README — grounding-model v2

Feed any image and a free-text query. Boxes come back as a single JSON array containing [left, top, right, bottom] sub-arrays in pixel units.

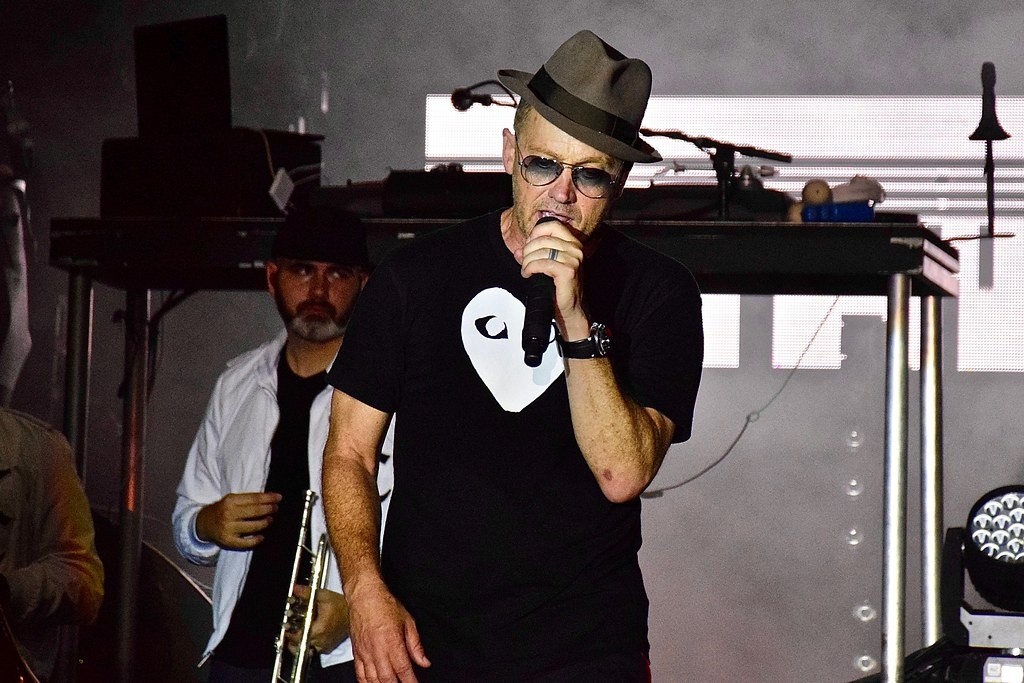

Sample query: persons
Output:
[[323, 29, 704, 683], [174, 204, 397, 683], [0, 411, 110, 683]]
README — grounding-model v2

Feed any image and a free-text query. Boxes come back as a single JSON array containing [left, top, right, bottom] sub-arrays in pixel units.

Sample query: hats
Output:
[[496, 29, 663, 163], [271, 205, 369, 271]]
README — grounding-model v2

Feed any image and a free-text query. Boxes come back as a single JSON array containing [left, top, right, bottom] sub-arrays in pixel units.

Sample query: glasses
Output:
[[514, 134, 628, 199]]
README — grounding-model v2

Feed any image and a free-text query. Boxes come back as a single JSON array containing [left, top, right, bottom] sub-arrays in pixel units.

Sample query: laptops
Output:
[[131, 14, 326, 145]]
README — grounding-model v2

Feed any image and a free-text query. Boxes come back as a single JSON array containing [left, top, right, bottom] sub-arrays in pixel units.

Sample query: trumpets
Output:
[[270, 490, 332, 683]]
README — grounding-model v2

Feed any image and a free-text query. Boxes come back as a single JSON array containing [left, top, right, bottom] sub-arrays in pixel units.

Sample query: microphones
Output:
[[452, 88, 493, 111], [521, 216, 564, 368]]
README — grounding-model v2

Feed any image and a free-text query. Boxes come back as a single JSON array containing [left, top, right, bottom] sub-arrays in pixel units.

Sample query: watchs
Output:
[[553, 322, 614, 360]]
